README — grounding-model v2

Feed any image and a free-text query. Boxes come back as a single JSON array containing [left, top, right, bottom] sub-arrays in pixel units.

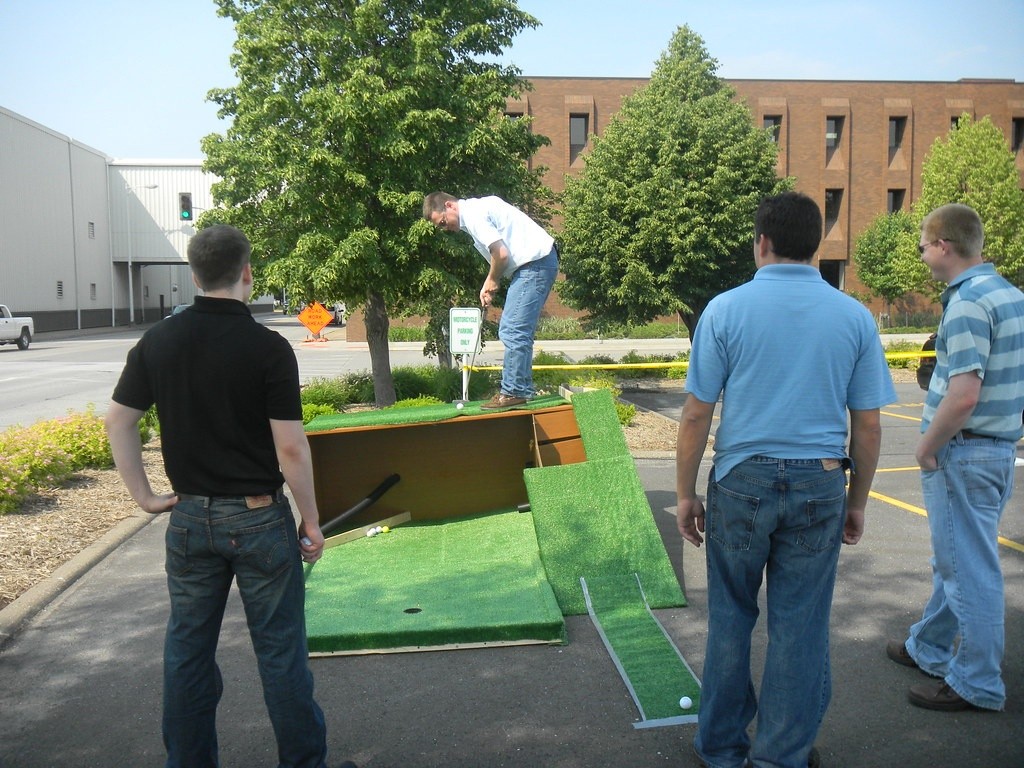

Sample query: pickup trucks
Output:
[[0, 304, 34, 350]]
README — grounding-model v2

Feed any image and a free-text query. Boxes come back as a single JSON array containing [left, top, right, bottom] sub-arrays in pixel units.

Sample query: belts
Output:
[[951, 428, 997, 443], [173, 491, 246, 503]]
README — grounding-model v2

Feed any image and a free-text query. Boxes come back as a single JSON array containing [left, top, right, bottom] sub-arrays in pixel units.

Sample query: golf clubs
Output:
[[450, 301, 489, 407]]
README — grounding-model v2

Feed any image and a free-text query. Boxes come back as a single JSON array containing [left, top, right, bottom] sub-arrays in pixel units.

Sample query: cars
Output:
[[321, 303, 344, 326], [164, 305, 191, 320], [283, 299, 305, 315]]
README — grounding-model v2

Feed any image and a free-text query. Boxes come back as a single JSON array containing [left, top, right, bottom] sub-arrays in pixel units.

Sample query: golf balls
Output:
[[456, 403, 464, 410], [366, 524, 391, 538], [678, 696, 693, 710]]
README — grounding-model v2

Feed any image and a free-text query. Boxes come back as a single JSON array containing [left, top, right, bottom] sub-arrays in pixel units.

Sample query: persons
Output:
[[886, 204, 1024, 712], [423, 191, 559, 410], [676, 192, 896, 768], [105, 224, 357, 768]]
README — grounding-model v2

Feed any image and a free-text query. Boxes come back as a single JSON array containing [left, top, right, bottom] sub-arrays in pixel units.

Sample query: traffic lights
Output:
[[179, 193, 192, 221]]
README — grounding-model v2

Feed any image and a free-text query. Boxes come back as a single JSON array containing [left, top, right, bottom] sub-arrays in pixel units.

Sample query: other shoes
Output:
[[805, 746, 821, 767]]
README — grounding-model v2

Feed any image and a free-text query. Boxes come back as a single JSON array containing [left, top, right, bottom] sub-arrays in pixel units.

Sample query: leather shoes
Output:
[[908, 677, 999, 712], [886, 638, 925, 667]]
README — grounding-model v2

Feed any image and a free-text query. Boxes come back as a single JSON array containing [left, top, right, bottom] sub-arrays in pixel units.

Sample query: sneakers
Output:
[[481, 393, 527, 410]]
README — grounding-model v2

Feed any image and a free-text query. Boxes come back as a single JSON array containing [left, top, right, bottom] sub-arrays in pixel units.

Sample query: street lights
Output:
[[126, 183, 158, 326]]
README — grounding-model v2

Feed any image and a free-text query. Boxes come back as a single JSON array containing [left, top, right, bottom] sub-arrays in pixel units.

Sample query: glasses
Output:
[[918, 238, 959, 253], [434, 209, 446, 229]]
